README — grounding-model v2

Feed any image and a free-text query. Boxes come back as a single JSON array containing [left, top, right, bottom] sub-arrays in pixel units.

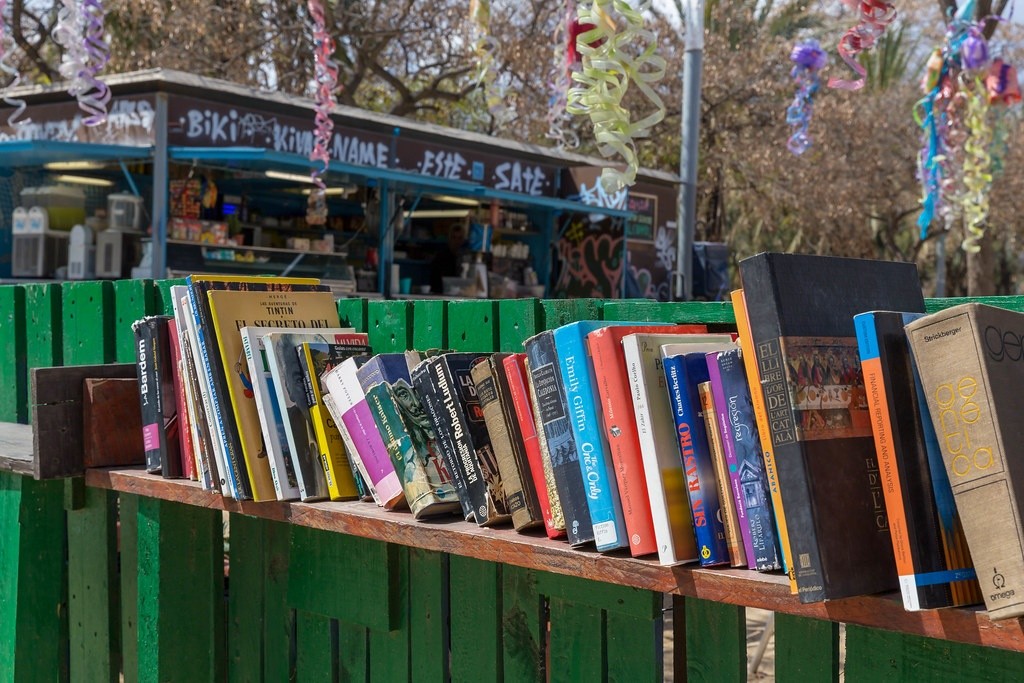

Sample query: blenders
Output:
[[96, 193, 146, 280]]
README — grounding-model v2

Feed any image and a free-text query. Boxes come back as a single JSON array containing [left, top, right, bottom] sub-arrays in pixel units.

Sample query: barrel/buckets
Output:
[[400, 277, 411, 294], [421, 285, 430, 293]]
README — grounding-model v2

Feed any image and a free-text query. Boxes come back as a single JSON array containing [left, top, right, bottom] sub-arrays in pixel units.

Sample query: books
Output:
[[740, 252, 925, 605], [904, 301, 1023, 622], [150, 275, 379, 504], [133, 316, 165, 472], [854, 310, 988, 611], [353, 350, 462, 522], [321, 355, 404, 511], [410, 290, 799, 596]]
